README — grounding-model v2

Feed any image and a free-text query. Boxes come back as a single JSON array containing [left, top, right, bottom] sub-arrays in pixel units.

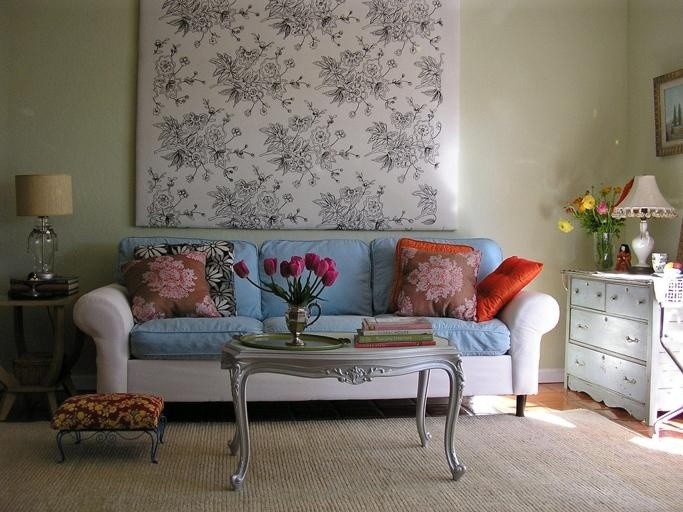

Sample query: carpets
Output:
[[0, 407, 683, 512]]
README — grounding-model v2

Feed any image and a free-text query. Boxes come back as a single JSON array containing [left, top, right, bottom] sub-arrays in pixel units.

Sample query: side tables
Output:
[[0, 291, 85, 420]]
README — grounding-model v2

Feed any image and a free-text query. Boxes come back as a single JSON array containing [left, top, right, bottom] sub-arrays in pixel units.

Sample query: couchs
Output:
[[73, 236, 561, 417]]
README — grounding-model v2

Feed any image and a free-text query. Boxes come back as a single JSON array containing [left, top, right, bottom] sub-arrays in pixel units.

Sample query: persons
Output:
[[616, 244, 632, 272]]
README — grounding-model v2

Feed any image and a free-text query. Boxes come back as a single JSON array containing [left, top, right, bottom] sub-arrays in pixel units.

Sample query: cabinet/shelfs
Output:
[[561, 267, 682, 427]]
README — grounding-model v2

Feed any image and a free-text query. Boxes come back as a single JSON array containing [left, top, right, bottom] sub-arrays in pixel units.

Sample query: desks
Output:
[[221, 332, 467, 491]]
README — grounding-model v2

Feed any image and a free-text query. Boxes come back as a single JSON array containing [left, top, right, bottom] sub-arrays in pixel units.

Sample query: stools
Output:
[[51, 392, 167, 464]]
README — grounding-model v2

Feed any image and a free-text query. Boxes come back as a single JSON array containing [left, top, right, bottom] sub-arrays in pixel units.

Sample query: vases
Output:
[[284, 302, 321, 346], [592, 233, 617, 272]]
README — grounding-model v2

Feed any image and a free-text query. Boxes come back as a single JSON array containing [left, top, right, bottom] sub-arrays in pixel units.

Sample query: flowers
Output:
[[557, 183, 626, 239], [233, 253, 339, 306]]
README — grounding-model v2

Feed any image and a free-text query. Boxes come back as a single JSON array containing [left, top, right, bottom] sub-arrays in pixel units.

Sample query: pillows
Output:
[[133, 240, 238, 317], [116, 250, 223, 324], [385, 238, 544, 323]]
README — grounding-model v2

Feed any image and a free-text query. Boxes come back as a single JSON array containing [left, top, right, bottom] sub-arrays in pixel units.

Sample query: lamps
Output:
[[8, 173, 80, 280], [611, 175, 679, 268]]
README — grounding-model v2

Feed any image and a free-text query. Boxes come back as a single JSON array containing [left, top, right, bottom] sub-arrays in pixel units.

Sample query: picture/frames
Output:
[[653, 69, 683, 157]]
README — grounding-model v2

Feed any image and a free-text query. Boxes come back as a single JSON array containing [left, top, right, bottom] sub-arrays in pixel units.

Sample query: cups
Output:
[[652, 253, 668, 273]]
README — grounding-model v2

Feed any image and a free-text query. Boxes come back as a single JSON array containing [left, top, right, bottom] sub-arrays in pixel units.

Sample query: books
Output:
[[354, 317, 436, 348]]
